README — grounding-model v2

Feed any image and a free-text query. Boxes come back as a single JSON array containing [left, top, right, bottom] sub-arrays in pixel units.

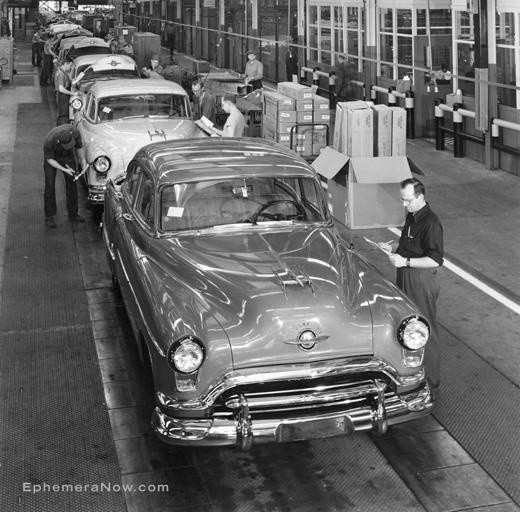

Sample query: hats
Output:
[[58, 130, 76, 150]]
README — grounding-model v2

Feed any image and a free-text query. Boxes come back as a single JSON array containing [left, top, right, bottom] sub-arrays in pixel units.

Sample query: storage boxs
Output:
[[260, 81, 427, 231]]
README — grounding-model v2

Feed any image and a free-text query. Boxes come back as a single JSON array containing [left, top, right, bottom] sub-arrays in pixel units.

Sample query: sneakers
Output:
[[45, 217, 55, 227], [68, 214, 85, 222]]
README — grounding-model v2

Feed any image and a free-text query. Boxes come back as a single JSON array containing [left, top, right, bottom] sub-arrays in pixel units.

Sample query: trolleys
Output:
[[289, 124, 329, 165], [216, 83, 254, 126]]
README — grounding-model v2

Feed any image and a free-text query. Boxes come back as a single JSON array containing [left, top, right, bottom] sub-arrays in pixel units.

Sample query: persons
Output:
[[241, 50, 263, 93], [333, 52, 358, 123], [44, 124, 87, 228], [32, 27, 219, 129], [210, 93, 246, 137], [378, 178, 444, 338]]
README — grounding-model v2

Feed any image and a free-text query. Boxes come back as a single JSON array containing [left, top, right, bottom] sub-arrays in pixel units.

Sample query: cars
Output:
[[232, 6, 294, 61], [67, 53, 143, 121], [72, 78, 211, 224], [102, 137, 435, 453], [37, 10, 108, 89]]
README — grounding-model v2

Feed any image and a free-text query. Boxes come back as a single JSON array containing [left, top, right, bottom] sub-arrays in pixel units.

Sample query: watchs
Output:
[[405, 257, 410, 267]]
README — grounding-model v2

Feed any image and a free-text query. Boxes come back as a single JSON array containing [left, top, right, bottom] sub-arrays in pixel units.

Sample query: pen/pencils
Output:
[[385, 240, 394, 244]]
